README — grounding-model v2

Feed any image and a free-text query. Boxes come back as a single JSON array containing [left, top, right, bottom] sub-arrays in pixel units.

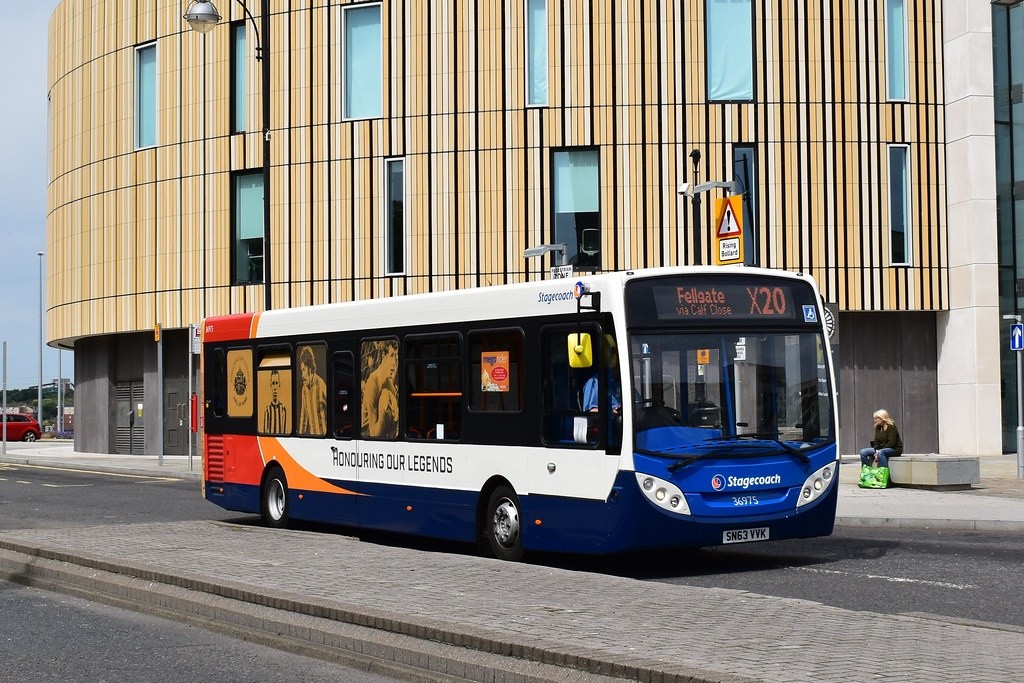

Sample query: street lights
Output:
[[183, 0, 273, 312], [38, 252, 45, 435]]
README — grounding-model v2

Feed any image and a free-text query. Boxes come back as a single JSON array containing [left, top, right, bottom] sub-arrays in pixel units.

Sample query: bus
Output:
[[198, 267, 845, 569]]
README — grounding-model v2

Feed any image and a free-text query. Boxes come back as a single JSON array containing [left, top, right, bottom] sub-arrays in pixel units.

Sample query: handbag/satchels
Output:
[[858, 464, 891, 489]]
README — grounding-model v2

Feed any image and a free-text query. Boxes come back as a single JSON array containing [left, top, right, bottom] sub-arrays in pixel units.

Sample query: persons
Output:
[[361, 341, 399, 438], [361, 349, 379, 391], [264, 370, 287, 434], [583, 346, 643, 439], [299, 346, 327, 435], [860, 408, 904, 489]]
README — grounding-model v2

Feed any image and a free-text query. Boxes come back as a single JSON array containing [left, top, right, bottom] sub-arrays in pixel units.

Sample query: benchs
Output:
[[873, 453, 980, 491]]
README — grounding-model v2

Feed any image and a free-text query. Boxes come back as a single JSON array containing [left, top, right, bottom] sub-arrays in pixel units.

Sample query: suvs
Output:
[[0, 412, 42, 443]]
